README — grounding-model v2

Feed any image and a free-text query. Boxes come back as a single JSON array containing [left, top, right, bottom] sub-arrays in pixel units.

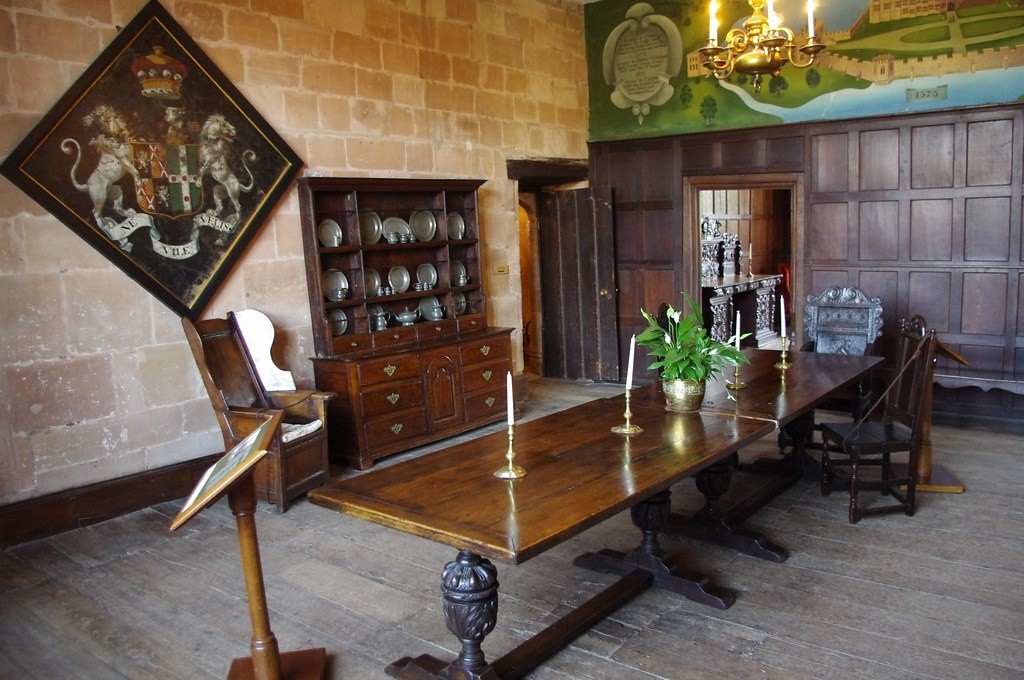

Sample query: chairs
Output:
[[180, 309, 338, 513], [777, 285, 884, 453], [820, 317, 937, 524]]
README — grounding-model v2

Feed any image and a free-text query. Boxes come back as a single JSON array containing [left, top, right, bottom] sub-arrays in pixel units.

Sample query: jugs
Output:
[[458, 275, 469, 286], [431, 304, 443, 320]]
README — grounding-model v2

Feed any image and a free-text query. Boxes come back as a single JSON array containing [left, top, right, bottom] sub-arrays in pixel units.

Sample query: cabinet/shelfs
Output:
[[295, 175, 518, 469], [699, 217, 785, 352]]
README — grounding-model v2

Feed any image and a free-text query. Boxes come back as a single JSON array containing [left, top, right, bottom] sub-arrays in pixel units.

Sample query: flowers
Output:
[[633, 290, 753, 390]]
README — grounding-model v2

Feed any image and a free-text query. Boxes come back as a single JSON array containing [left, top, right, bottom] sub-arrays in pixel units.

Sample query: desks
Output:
[[306, 352, 883, 678]]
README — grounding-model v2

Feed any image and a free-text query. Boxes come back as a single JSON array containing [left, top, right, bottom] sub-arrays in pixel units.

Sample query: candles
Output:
[[699, 0, 827, 93], [505, 369, 516, 429], [624, 334, 637, 391]]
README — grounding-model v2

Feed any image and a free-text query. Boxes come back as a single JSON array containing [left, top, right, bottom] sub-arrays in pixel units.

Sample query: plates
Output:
[[416, 263, 437, 286], [322, 272, 349, 301], [409, 205, 436, 241], [364, 267, 381, 295], [450, 260, 466, 284], [318, 218, 343, 247], [447, 211, 465, 240], [326, 308, 348, 336], [452, 292, 466, 316], [358, 208, 382, 244], [419, 296, 439, 320], [388, 266, 410, 293], [382, 217, 412, 241], [366, 304, 383, 330]]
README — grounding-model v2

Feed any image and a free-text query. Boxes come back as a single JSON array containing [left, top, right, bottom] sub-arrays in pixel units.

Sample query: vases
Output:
[[660, 371, 707, 414]]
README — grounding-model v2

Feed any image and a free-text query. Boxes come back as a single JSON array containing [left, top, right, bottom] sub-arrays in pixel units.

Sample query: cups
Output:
[[384, 286, 391, 296], [391, 287, 396, 294], [409, 233, 416, 243], [376, 287, 384, 296], [387, 232, 398, 244], [331, 236, 342, 248], [400, 234, 407, 243], [422, 282, 428, 290], [429, 283, 433, 290]]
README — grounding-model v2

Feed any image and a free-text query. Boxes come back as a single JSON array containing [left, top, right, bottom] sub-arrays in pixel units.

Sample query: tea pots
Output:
[[413, 282, 422, 291], [374, 311, 391, 331], [392, 306, 422, 327], [335, 287, 348, 301]]
